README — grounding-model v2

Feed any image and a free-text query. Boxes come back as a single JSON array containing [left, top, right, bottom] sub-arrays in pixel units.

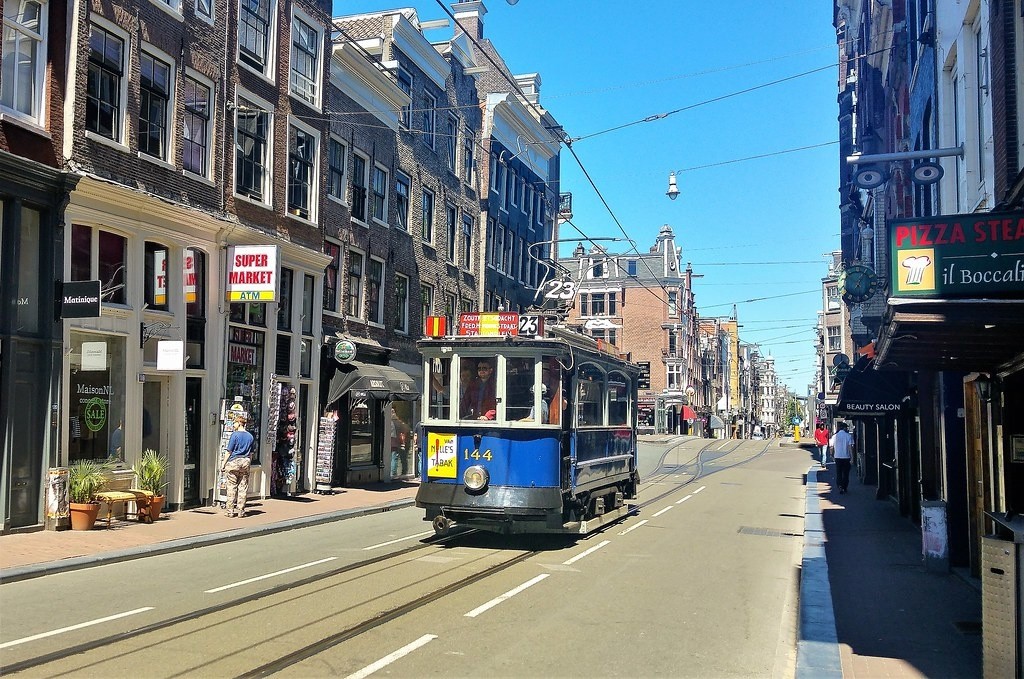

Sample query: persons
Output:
[[519, 383, 550, 424], [389, 409, 424, 479], [430, 363, 476, 411], [111, 419, 122, 459], [457, 359, 498, 422], [828, 423, 855, 494], [814, 424, 829, 468], [220, 414, 254, 517]]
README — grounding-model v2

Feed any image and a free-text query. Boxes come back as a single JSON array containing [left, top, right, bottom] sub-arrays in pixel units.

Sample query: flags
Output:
[[324, 355, 420, 410]]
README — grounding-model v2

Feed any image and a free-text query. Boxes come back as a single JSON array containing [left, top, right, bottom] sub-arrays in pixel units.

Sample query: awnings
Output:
[[682, 406, 698, 420], [708, 415, 725, 429], [836, 353, 920, 419]]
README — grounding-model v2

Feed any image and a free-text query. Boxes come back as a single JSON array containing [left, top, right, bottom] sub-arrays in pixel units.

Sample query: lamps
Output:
[[666, 170, 681, 201], [974, 373, 992, 402]]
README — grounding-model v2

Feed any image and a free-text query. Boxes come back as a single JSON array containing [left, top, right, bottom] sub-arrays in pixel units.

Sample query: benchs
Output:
[[94, 488, 155, 531]]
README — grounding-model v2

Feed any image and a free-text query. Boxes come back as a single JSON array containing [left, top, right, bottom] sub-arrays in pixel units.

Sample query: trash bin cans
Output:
[[978, 510, 1024, 679]]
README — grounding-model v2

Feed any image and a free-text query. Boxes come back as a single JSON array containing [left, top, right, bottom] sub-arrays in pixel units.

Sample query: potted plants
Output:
[[65, 457, 115, 530], [120, 448, 174, 520]]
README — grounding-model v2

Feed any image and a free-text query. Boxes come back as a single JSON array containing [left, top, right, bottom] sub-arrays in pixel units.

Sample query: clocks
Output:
[[837, 265, 878, 302]]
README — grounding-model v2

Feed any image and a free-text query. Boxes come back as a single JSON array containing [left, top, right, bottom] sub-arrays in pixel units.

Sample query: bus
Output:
[[414, 237, 646, 539]]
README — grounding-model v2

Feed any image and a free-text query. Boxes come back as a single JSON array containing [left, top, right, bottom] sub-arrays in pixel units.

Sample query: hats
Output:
[[841, 423, 849, 429], [529, 383, 547, 394]]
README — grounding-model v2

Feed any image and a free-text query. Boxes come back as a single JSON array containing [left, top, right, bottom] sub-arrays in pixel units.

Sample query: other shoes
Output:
[[224, 512, 234, 517], [238, 513, 247, 518], [839, 487, 845, 494], [821, 464, 825, 468]]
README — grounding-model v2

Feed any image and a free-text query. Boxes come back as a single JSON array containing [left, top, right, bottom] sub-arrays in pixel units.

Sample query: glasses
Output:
[[477, 366, 492, 372]]
